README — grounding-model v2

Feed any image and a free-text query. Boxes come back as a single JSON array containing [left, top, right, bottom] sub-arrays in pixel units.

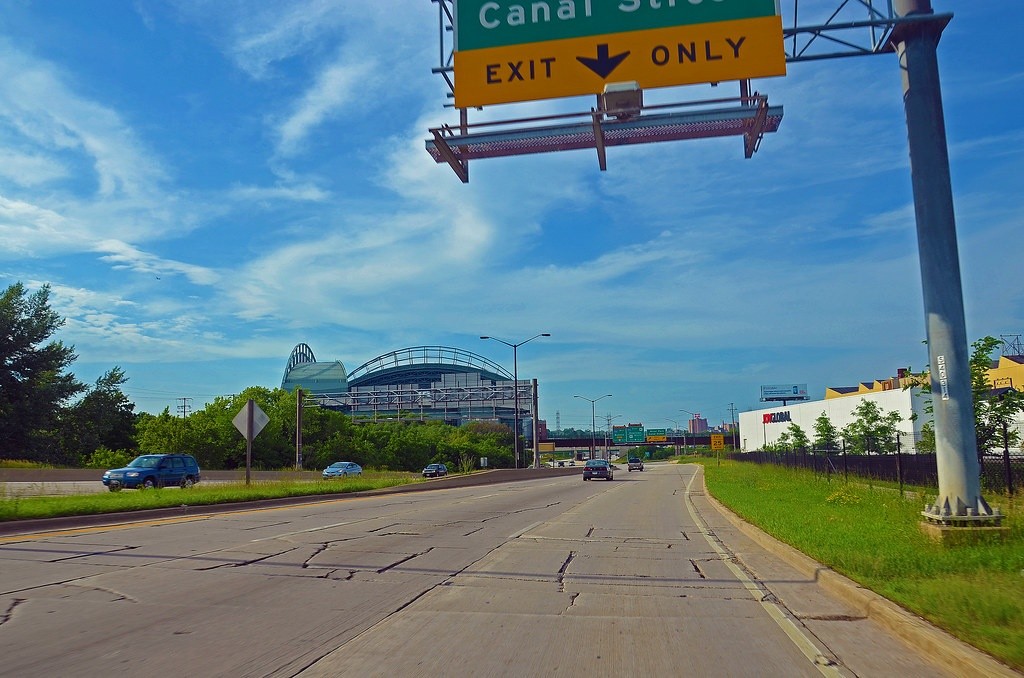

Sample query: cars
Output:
[[558, 462, 565, 467], [628, 457, 644, 472], [322, 462, 363, 480], [582, 459, 613, 481], [569, 460, 576, 466], [422, 464, 448, 478]]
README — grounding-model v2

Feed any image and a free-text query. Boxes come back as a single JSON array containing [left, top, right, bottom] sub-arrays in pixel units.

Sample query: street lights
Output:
[[596, 414, 623, 468], [574, 394, 612, 459], [679, 409, 696, 447], [665, 417, 678, 457], [480, 333, 551, 467]]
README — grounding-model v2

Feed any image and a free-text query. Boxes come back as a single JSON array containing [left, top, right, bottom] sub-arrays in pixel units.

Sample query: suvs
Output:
[[101, 452, 201, 492]]
